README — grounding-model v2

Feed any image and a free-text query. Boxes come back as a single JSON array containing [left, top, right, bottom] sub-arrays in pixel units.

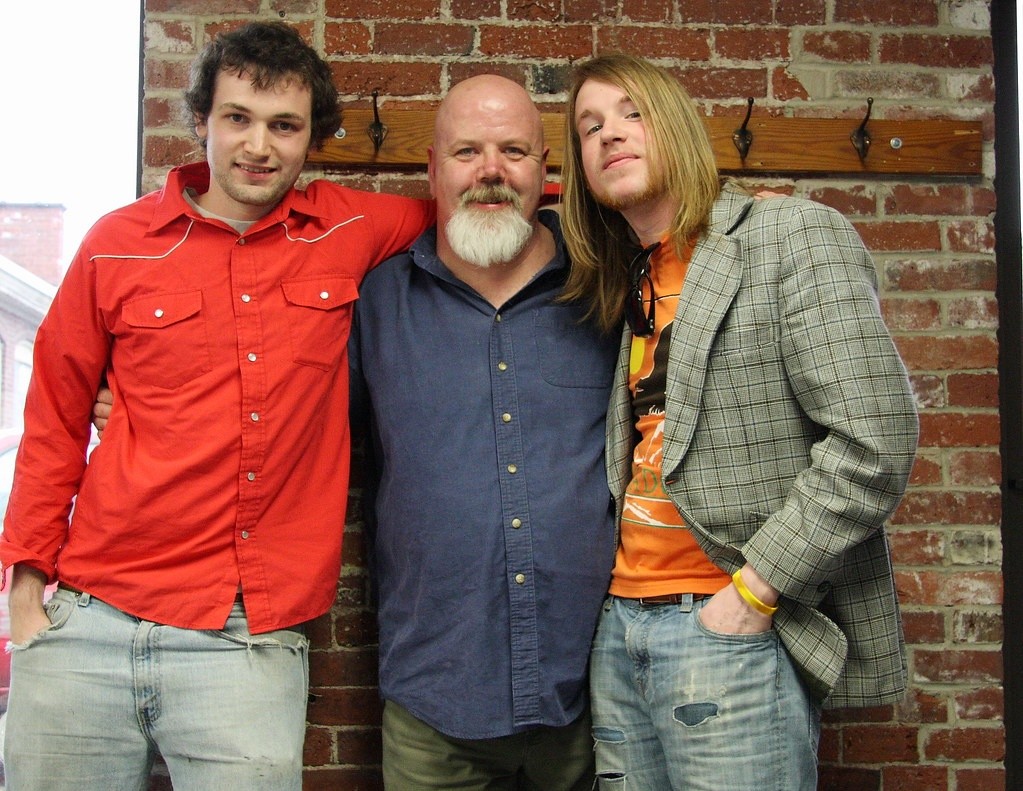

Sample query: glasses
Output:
[[622, 242, 662, 337]]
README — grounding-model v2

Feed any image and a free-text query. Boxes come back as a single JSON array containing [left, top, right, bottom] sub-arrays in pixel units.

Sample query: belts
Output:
[[615, 592, 715, 606]]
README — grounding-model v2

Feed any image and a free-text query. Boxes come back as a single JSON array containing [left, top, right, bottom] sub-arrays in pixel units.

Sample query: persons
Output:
[[558, 54, 919, 791], [92, 73, 779, 791], [0, 20, 567, 791]]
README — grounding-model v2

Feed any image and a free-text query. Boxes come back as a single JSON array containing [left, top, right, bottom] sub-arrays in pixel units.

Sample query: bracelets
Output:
[[731, 569, 779, 616]]
